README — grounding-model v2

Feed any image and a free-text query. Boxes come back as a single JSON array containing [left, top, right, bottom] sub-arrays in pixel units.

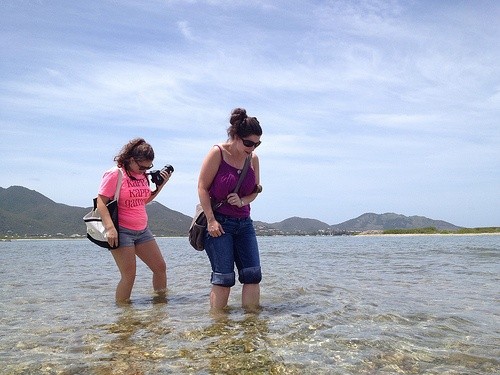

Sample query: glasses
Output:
[[135, 161, 153, 170], [240, 137, 261, 148]]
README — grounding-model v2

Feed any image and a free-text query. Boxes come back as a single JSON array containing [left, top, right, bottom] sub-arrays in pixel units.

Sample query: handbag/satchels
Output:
[[82, 169, 122, 249], [189, 200, 224, 251]]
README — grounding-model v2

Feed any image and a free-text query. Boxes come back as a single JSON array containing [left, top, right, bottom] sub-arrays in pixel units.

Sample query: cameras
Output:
[[150, 165, 174, 186]]
